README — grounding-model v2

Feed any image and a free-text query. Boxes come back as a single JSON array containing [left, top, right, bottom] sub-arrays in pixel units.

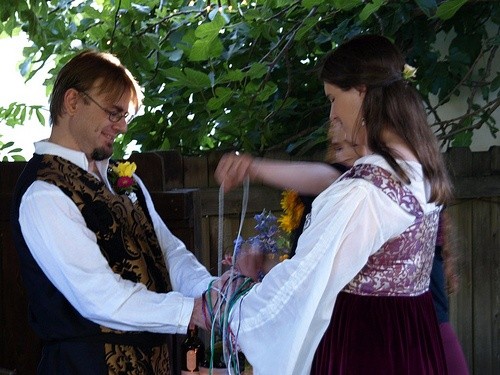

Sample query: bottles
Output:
[[185, 323, 201, 373]]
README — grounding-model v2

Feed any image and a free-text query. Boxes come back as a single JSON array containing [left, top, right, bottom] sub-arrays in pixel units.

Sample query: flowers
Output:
[[243, 188, 303, 262], [111, 161, 137, 194]]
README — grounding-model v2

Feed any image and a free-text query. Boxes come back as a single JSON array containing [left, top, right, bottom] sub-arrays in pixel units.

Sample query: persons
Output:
[[10, 34, 467, 375]]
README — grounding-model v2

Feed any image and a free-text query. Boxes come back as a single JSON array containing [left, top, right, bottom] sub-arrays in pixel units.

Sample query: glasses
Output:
[[80, 90, 134, 125]]
